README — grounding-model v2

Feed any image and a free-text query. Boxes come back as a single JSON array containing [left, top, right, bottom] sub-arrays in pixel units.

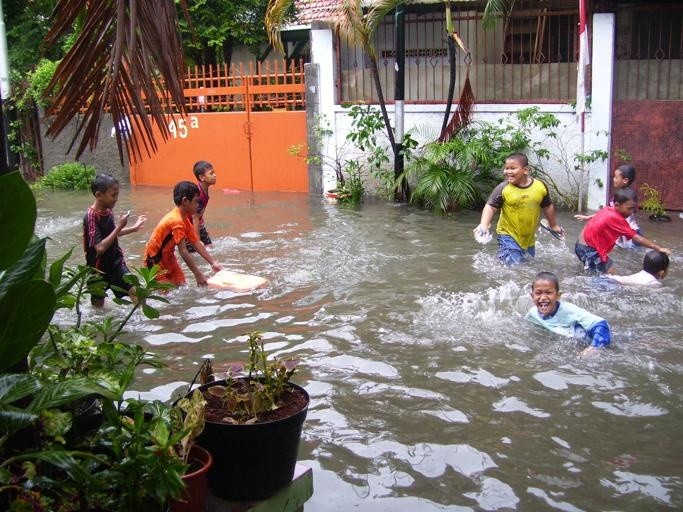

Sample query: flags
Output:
[[574, 1, 591, 123]]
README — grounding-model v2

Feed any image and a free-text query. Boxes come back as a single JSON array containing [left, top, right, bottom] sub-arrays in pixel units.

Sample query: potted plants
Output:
[[178, 330, 311, 500], [137, 389, 214, 510], [289, 104, 385, 206], [0, 170, 172, 509]]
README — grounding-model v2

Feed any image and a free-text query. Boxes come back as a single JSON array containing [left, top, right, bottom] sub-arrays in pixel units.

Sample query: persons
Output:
[[82, 174, 147, 310], [592, 250, 670, 295], [575, 188, 673, 275], [525, 272, 612, 360], [472, 153, 566, 266], [142, 180, 225, 298], [184, 160, 216, 253], [573, 164, 644, 248]]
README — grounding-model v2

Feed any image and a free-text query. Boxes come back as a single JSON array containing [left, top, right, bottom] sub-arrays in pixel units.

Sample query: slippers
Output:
[[472, 223, 490, 243], [540, 218, 566, 241]]
